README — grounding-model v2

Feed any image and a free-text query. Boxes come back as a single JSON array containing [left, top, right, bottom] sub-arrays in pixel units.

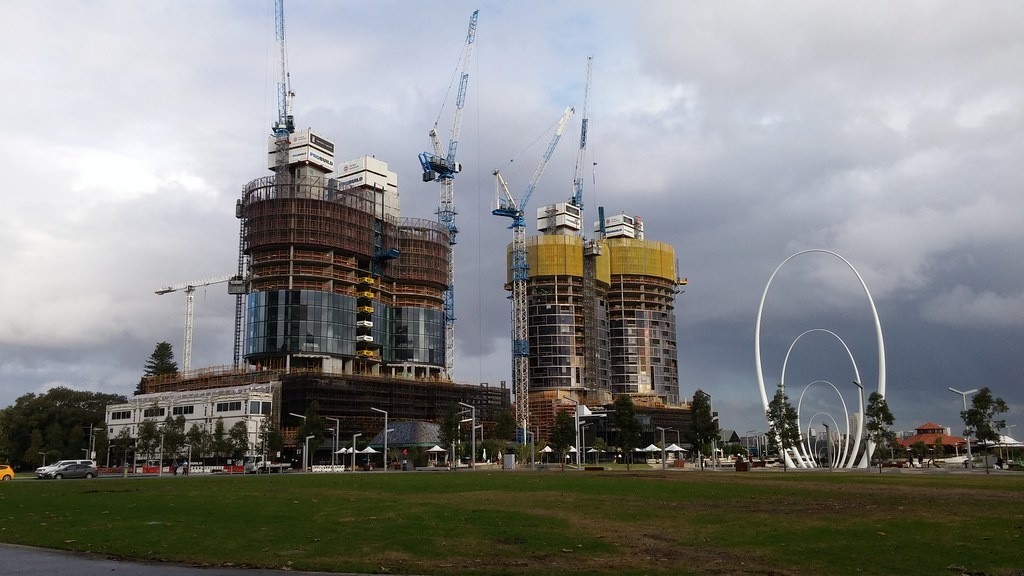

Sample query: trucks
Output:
[[245, 454, 292, 474]]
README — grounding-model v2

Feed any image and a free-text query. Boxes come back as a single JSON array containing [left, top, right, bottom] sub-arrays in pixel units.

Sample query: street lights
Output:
[[305, 435, 315, 472], [81, 448, 88, 460], [656, 426, 666, 473], [458, 401, 476, 472], [328, 428, 335, 471], [184, 443, 192, 476], [289, 412, 307, 427], [326, 417, 339, 465], [352, 433, 363, 471], [852, 379, 871, 469], [948, 387, 978, 471], [370, 407, 387, 470], [38, 452, 45, 478], [107, 444, 117, 468], [250, 419, 258, 454], [821, 421, 833, 472]]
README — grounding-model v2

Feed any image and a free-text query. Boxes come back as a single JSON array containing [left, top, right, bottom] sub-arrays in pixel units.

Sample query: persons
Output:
[[816, 458, 821, 468], [172, 458, 178, 476], [182, 460, 188, 475], [918, 453, 923, 464], [996, 457, 1003, 467], [737, 453, 743, 462], [716, 456, 721, 468], [909, 454, 915, 468], [821, 457, 824, 465], [749, 453, 753, 468]]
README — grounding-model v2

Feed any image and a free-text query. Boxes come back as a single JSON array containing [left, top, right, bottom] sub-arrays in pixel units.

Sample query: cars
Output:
[[0, 465, 15, 481], [45, 464, 98, 480]]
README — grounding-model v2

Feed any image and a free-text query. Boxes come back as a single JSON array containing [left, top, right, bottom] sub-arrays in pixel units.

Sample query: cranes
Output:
[[274, 0, 296, 198], [155, 275, 233, 392], [418, 9, 478, 383], [490, 107, 575, 445], [567, 56, 593, 208]]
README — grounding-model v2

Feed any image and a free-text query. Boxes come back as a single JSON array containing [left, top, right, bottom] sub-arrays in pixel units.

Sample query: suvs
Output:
[[35, 459, 97, 479]]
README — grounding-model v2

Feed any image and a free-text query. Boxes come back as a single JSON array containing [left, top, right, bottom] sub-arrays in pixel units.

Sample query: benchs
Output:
[[705, 460, 736, 468]]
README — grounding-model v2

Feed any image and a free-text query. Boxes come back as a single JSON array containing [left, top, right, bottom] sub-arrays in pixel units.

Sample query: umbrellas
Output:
[[977, 435, 1024, 463], [630, 443, 688, 459], [565, 446, 582, 463], [333, 446, 382, 465], [586, 448, 606, 460], [425, 445, 448, 451], [539, 446, 555, 463]]
